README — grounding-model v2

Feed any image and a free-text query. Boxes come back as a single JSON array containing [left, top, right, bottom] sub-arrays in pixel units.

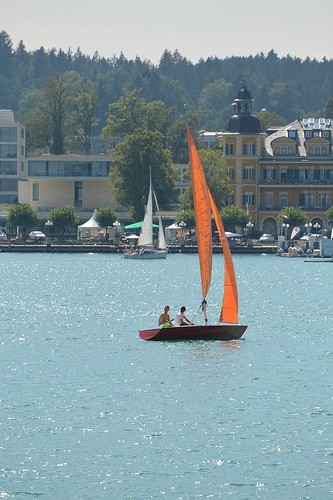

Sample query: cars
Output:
[[28, 231, 46, 241], [256, 234, 275, 245]]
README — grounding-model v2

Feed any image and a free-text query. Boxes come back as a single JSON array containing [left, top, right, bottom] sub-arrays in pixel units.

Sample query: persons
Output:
[[275, 247, 284, 256], [176, 306, 194, 326], [288, 246, 304, 256], [158, 306, 174, 327], [116, 240, 121, 246], [123, 247, 145, 256], [306, 248, 313, 255]]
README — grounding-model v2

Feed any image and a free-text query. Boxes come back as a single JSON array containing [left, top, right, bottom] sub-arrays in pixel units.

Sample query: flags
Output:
[[291, 227, 300, 240]]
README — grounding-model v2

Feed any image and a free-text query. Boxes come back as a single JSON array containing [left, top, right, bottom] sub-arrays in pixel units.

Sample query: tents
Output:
[[164, 222, 185, 242], [77, 208, 114, 243], [123, 221, 159, 244]]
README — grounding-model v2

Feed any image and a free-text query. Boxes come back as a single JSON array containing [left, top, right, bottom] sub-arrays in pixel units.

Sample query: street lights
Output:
[[113, 220, 121, 246], [304, 222, 313, 236], [313, 222, 321, 237], [281, 222, 290, 240], [178, 221, 187, 247], [45, 220, 53, 247], [246, 221, 254, 248]]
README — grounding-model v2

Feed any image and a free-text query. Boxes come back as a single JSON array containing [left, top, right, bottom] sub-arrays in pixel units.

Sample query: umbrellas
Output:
[[125, 234, 140, 239], [217, 231, 243, 239]]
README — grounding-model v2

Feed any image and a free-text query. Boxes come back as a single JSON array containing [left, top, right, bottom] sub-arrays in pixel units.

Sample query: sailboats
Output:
[[137, 123, 248, 342], [122, 165, 168, 260]]
